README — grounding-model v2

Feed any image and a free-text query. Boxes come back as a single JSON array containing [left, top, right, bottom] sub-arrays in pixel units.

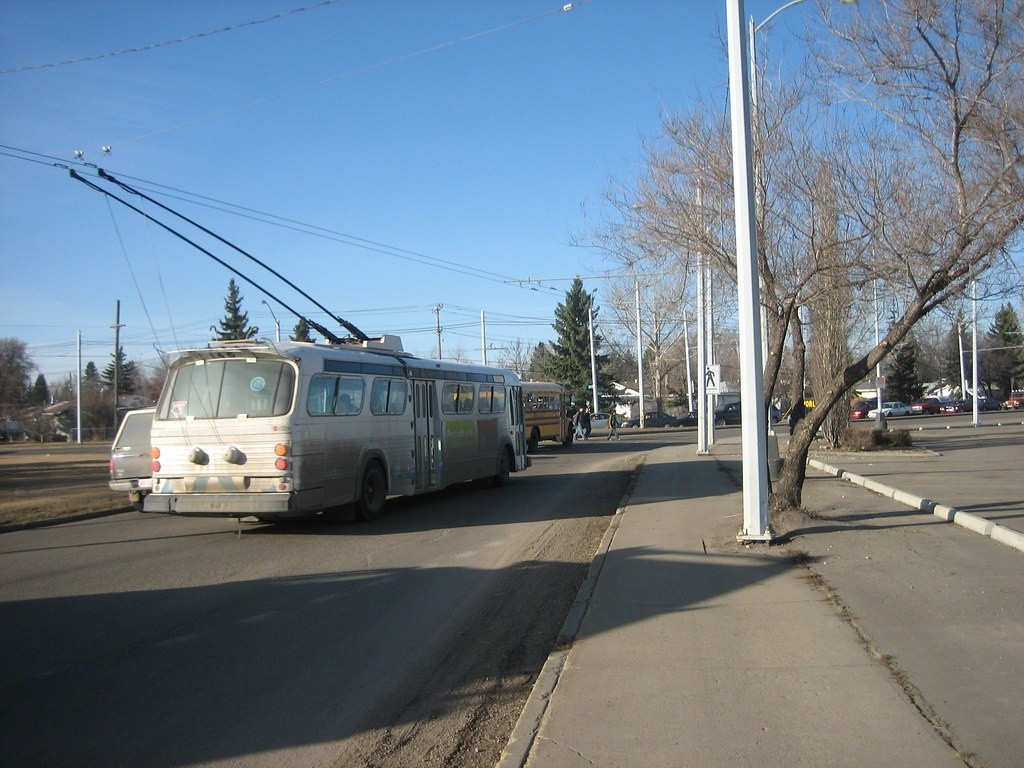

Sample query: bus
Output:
[[453, 380, 575, 455], [68, 163, 534, 524]]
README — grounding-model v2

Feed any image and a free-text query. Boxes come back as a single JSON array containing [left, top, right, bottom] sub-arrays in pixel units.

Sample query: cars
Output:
[[868, 401, 914, 419], [938, 389, 1024, 416], [621, 410, 678, 430], [850, 400, 875, 420], [671, 411, 698, 428], [712, 399, 781, 427], [578, 413, 623, 438], [909, 396, 951, 415]]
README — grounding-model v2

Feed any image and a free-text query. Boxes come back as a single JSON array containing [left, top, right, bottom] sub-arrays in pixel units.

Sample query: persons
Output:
[[787, 398, 806, 446], [606, 410, 621, 441], [572, 400, 596, 441], [337, 394, 358, 413], [442, 396, 501, 412]]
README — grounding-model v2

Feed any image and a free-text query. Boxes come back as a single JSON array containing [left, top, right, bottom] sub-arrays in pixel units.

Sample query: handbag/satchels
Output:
[[607, 421, 610, 429]]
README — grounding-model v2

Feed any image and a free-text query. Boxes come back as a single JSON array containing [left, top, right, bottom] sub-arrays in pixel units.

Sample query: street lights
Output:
[[588, 287, 598, 418], [261, 299, 281, 341], [634, 179, 710, 456]]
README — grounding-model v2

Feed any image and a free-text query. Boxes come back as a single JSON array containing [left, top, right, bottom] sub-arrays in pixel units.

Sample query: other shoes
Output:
[[617, 438, 621, 441], [607, 438, 611, 441]]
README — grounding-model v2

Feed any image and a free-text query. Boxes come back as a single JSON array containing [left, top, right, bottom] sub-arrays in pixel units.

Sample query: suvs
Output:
[[108, 409, 158, 513]]
